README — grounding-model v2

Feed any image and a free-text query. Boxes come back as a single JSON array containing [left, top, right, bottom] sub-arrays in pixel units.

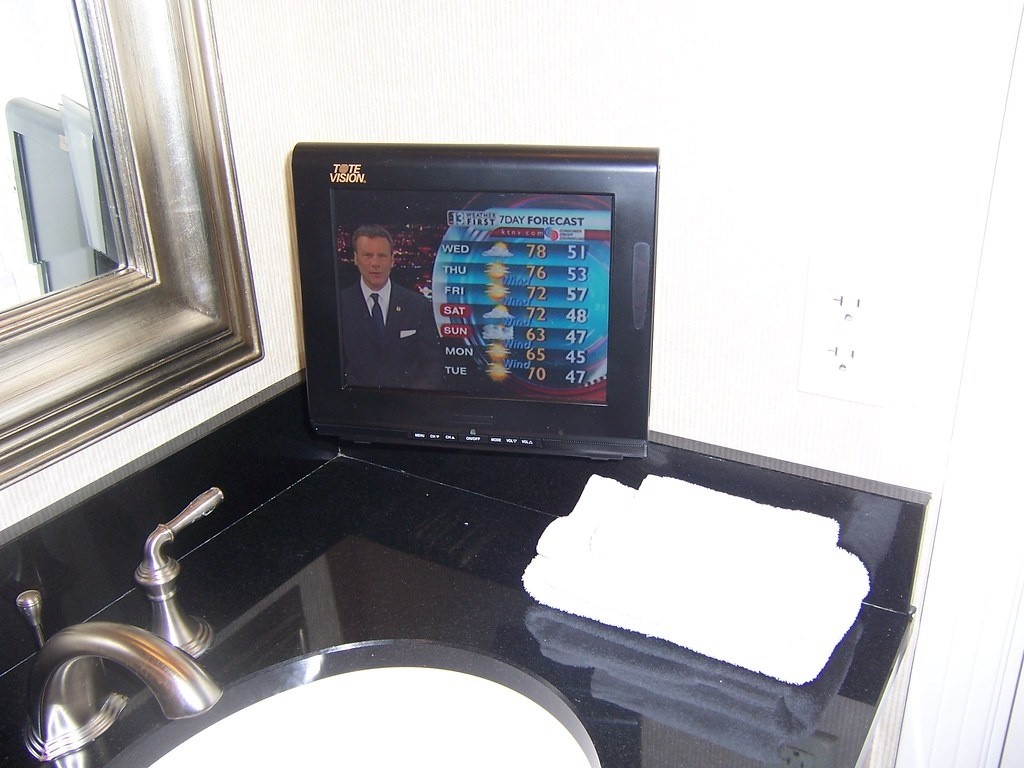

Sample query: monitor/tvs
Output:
[[289, 142, 658, 458]]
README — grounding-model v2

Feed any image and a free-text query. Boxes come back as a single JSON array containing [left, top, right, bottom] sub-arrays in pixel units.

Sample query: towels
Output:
[[520, 472, 870, 685]]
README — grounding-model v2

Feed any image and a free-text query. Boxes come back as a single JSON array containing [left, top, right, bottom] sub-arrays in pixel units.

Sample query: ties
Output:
[[370, 293, 384, 341]]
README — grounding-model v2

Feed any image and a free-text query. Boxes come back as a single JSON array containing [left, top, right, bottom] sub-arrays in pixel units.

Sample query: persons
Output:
[[338, 226, 443, 390]]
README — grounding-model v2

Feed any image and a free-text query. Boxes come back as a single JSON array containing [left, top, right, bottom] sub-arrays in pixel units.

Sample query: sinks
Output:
[[104, 639, 603, 768]]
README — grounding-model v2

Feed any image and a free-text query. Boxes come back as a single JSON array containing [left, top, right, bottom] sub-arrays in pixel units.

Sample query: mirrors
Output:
[[0, 0, 265, 486]]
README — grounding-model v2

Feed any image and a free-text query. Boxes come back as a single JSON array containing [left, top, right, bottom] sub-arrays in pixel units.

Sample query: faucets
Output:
[[23, 622, 225, 761]]
